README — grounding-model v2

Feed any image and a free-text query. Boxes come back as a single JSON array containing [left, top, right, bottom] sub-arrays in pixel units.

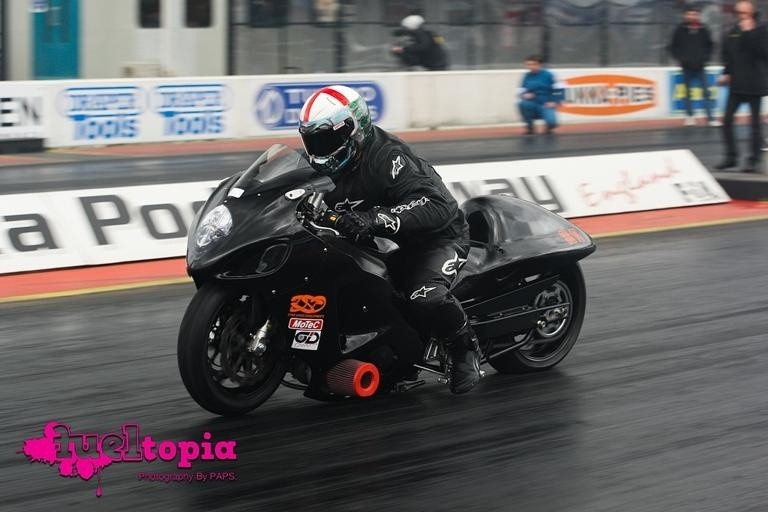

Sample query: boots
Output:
[[444, 316, 482, 393]]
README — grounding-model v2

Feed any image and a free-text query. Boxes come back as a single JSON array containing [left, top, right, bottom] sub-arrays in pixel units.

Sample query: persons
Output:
[[666, 8, 722, 127], [385, 13, 449, 72], [514, 53, 559, 135], [297, 81, 487, 395], [712, 2, 767, 175]]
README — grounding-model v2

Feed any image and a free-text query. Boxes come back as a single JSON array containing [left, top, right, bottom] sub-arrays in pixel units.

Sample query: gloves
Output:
[[337, 210, 375, 240]]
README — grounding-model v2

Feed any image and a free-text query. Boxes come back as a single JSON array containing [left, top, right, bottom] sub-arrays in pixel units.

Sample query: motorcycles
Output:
[[174, 140, 600, 418]]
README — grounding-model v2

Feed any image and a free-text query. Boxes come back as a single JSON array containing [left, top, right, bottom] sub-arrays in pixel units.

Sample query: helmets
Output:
[[299, 84, 374, 179], [401, 13, 424, 29]]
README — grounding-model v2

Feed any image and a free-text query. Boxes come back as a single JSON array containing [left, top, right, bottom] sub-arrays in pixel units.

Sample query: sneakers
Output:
[[685, 116, 696, 126], [714, 160, 737, 170], [743, 157, 759, 170], [708, 118, 724, 127]]
